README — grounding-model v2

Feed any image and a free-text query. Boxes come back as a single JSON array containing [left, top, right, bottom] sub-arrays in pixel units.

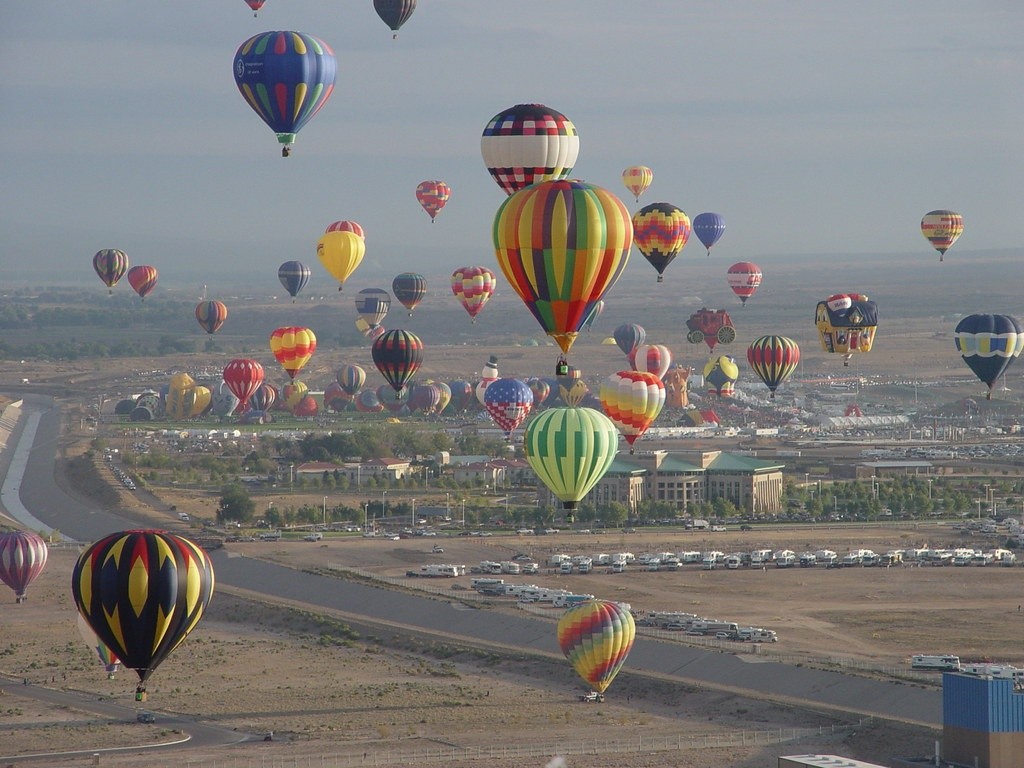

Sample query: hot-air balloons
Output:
[[88, 306, 801, 421], [91, 249, 130, 295], [622, 166, 653, 203], [415, 178, 451, 223], [276, 260, 311, 303], [245, 0, 268, 18], [316, 231, 367, 292], [481, 377, 533, 442], [481, 102, 581, 202], [693, 212, 726, 256], [492, 178, 639, 377], [231, 31, 338, 159], [595, 369, 668, 453], [727, 262, 762, 308], [325, 220, 365, 248], [73, 529, 215, 703], [193, 300, 227, 332], [127, 266, 159, 304], [75, 611, 120, 680], [523, 404, 620, 523], [390, 273, 428, 317], [955, 314, 1024, 401], [814, 293, 879, 366], [355, 288, 391, 338], [0, 530, 48, 605], [374, 0, 418, 40], [449, 266, 497, 323], [557, 598, 637, 703], [631, 202, 691, 283], [920, 210, 964, 262]]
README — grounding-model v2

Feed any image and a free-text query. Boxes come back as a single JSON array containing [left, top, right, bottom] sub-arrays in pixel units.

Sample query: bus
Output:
[[416, 515, 1023, 683]]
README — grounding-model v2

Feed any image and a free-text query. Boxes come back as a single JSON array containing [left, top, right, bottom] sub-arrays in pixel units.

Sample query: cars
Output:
[[162, 504, 413, 548], [99, 445, 136, 492]]
